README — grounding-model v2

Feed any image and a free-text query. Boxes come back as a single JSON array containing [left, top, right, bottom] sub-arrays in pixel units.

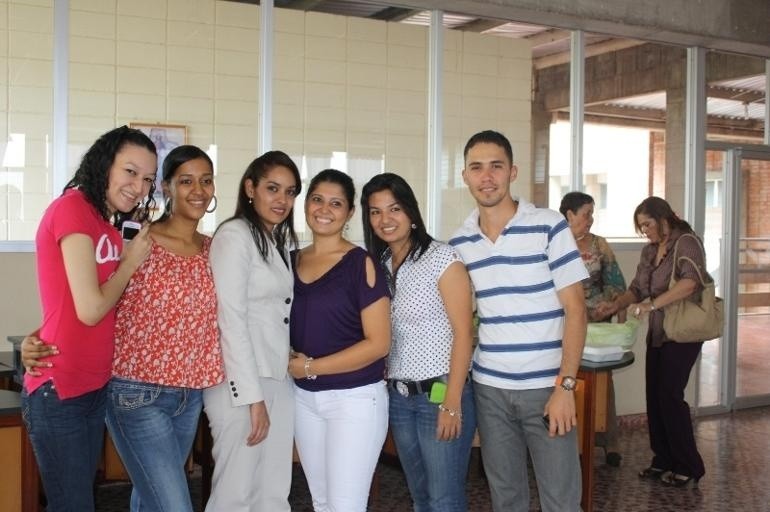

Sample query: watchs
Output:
[[554, 374, 578, 392]]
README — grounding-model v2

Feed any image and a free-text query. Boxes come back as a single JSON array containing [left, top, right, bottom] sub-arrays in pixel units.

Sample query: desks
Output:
[[0, 351, 16, 389], [0, 389, 38, 511], [7, 335, 25, 378], [573, 352, 635, 511]]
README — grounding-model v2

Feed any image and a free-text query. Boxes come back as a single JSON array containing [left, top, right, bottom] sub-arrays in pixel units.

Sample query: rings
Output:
[[634, 306, 642, 317]]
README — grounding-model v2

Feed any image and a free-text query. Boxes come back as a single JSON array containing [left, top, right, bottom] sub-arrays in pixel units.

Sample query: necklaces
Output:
[[575, 234, 587, 241]]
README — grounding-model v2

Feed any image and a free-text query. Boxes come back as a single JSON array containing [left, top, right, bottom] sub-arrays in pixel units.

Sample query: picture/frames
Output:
[[128, 121, 189, 211]]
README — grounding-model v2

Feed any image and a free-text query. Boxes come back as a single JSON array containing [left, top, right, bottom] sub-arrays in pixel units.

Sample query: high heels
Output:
[[639, 467, 666, 480], [666, 464, 705, 490]]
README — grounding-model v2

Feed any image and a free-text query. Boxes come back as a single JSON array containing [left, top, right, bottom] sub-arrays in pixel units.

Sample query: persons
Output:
[[287, 168, 393, 510], [20, 124, 159, 512], [446, 129, 591, 512], [198, 149, 303, 510], [19, 145, 219, 512], [359, 172, 478, 511], [589, 195, 707, 489], [556, 190, 628, 468]]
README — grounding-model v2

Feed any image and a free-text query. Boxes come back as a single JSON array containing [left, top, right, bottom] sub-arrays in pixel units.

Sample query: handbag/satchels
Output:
[[662, 273, 724, 343]]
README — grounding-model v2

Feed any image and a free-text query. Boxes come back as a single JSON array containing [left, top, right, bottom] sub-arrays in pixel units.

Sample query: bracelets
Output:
[[303, 356, 318, 381], [437, 404, 464, 419], [650, 298, 657, 312]]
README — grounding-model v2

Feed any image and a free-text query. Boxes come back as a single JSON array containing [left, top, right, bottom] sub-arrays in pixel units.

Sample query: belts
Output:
[[388, 374, 448, 398]]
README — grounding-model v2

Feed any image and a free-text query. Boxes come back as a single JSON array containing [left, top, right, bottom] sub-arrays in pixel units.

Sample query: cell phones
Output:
[[122, 221, 142, 241], [430, 381, 446, 405]]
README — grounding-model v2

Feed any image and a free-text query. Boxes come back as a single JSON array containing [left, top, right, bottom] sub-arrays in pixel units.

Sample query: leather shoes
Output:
[[606, 452, 621, 469]]
